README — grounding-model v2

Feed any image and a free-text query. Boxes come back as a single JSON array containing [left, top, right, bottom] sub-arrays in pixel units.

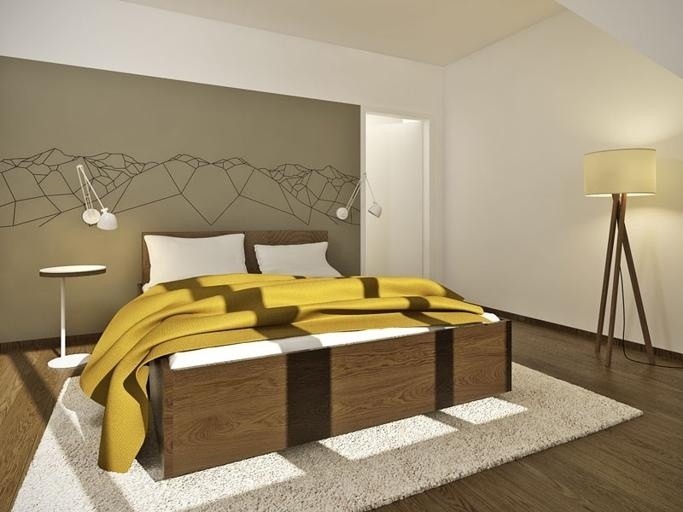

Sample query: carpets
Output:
[[10, 360, 644, 512]]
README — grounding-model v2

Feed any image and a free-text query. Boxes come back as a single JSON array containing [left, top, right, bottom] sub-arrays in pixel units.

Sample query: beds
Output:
[[137, 232, 512, 480]]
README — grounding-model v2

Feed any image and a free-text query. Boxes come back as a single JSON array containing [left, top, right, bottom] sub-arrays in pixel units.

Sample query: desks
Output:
[[39, 265, 106, 369]]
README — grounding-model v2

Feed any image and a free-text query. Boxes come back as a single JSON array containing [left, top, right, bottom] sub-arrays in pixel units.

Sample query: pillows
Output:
[[254, 241, 344, 275], [142, 234, 249, 293]]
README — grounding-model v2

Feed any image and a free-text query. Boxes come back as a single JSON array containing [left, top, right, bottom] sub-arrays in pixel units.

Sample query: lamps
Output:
[[76, 164, 118, 231], [582, 147, 657, 369], [336, 175, 382, 221]]
